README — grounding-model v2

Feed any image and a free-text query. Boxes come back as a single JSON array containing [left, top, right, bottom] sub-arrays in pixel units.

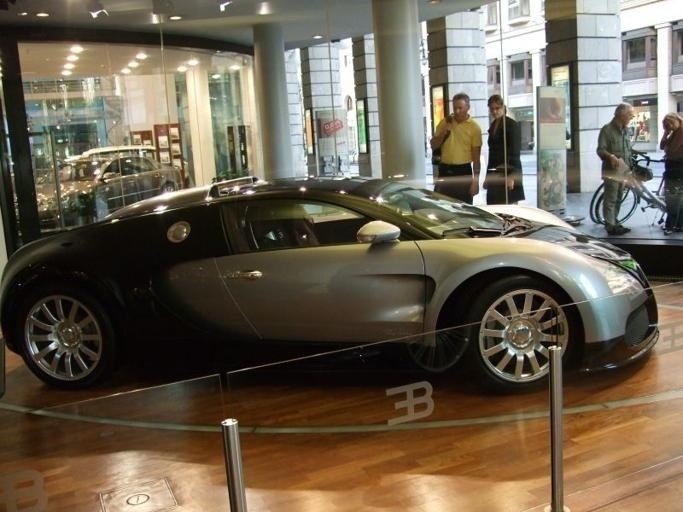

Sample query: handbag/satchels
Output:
[[431, 148, 441, 165], [633, 163, 654, 181]]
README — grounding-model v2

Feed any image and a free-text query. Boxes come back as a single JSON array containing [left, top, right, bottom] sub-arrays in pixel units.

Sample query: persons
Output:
[[482, 95, 527, 205], [430, 92, 482, 206], [596, 101, 648, 235], [659, 112, 682, 236]]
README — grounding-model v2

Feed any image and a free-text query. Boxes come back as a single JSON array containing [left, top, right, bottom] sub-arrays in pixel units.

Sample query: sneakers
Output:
[[605, 224, 630, 236]]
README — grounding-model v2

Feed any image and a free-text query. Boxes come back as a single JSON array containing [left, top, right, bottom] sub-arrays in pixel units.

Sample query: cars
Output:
[[12, 153, 183, 229]]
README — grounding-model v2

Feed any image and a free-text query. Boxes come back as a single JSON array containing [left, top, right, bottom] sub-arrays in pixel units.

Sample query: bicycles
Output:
[[590, 150, 667, 225]]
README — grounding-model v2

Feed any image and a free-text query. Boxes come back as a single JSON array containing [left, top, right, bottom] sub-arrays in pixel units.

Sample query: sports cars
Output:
[[0, 176, 659, 396]]
